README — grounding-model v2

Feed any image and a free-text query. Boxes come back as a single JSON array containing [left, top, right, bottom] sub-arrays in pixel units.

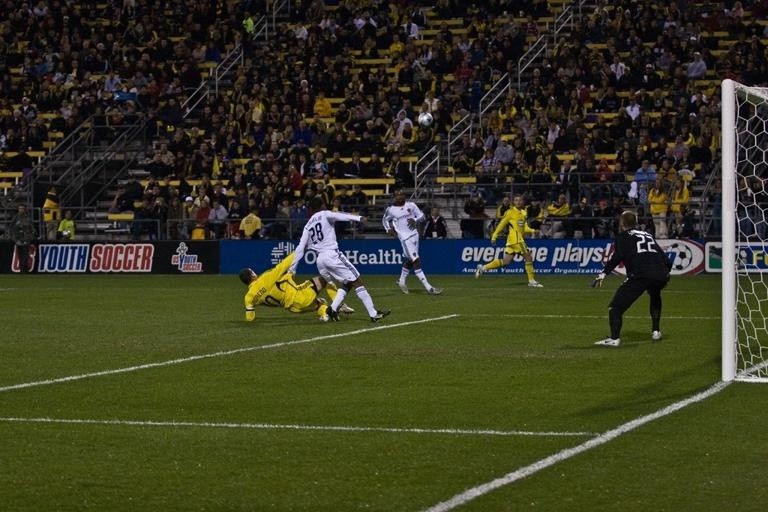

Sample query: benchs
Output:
[[0, 1, 765, 243]]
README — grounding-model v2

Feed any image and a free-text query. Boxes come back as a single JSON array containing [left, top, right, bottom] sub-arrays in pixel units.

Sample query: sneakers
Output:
[[475, 264, 483, 279], [371, 309, 391, 322], [593, 337, 621, 347], [338, 303, 354, 313], [652, 331, 662, 341], [528, 281, 543, 288], [426, 288, 444, 295], [326, 305, 340, 322], [396, 279, 409, 295]]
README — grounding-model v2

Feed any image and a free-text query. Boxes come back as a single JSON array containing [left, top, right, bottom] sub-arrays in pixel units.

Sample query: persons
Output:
[[591, 210, 672, 346], [9, 203, 36, 274], [381, 185, 443, 294], [239, 250, 354, 321], [286, 197, 392, 322], [1, 2, 767, 240], [475, 193, 544, 288]]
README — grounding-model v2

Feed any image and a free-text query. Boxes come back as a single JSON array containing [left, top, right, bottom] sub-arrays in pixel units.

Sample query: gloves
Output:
[[592, 273, 606, 288]]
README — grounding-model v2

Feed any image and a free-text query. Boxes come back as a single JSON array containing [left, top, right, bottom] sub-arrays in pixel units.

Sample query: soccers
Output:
[[417, 112, 432, 127]]
